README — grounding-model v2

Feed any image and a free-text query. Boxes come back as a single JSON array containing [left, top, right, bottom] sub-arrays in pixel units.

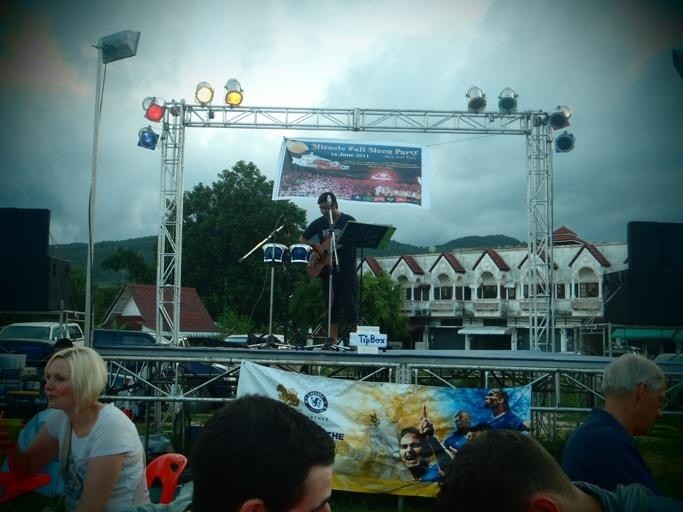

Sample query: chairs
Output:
[[145, 453, 188, 506]]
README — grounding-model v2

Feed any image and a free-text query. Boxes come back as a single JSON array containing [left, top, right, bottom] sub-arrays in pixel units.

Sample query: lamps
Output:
[[465, 85, 519, 116], [135, 96, 169, 150], [548, 104, 576, 154], [194, 78, 243, 108]]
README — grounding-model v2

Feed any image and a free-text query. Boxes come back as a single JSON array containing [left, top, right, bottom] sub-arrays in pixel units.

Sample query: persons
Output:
[[439, 354, 666, 511], [299, 192, 357, 350], [400, 389, 532, 481]]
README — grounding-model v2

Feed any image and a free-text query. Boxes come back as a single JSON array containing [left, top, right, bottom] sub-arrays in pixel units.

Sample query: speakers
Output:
[[327, 195, 332, 206], [602, 270, 628, 323], [0, 207, 50, 310], [626, 221, 683, 325], [48, 256, 72, 310]]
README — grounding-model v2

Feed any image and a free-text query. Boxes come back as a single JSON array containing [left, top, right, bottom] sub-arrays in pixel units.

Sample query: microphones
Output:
[[282, 217, 291, 238]]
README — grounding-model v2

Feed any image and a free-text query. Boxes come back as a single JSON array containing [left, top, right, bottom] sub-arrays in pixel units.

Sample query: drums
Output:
[[262, 243, 289, 264], [290, 244, 313, 265]]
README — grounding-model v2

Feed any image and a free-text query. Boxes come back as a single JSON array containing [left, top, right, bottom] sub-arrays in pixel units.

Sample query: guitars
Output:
[[307, 220, 352, 277]]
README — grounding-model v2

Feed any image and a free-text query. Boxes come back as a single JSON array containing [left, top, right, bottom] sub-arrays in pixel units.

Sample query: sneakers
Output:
[[321, 339, 358, 351]]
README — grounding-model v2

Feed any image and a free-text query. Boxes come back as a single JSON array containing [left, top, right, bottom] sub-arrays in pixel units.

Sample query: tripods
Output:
[[238, 224, 301, 349], [336, 246, 387, 352], [303, 206, 353, 350]]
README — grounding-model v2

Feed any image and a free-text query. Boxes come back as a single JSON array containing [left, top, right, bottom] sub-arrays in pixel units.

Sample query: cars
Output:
[[1, 320, 84, 347], [89, 326, 239, 413]]
